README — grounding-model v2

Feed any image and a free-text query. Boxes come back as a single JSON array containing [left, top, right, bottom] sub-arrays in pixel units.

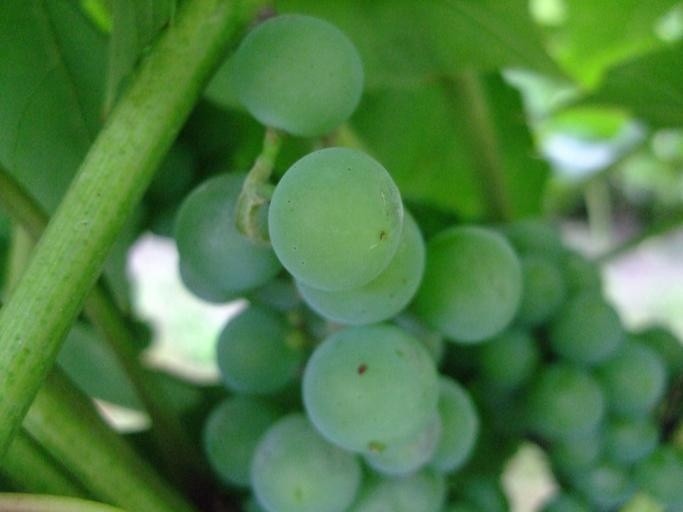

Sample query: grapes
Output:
[[173, 14, 683, 512]]
